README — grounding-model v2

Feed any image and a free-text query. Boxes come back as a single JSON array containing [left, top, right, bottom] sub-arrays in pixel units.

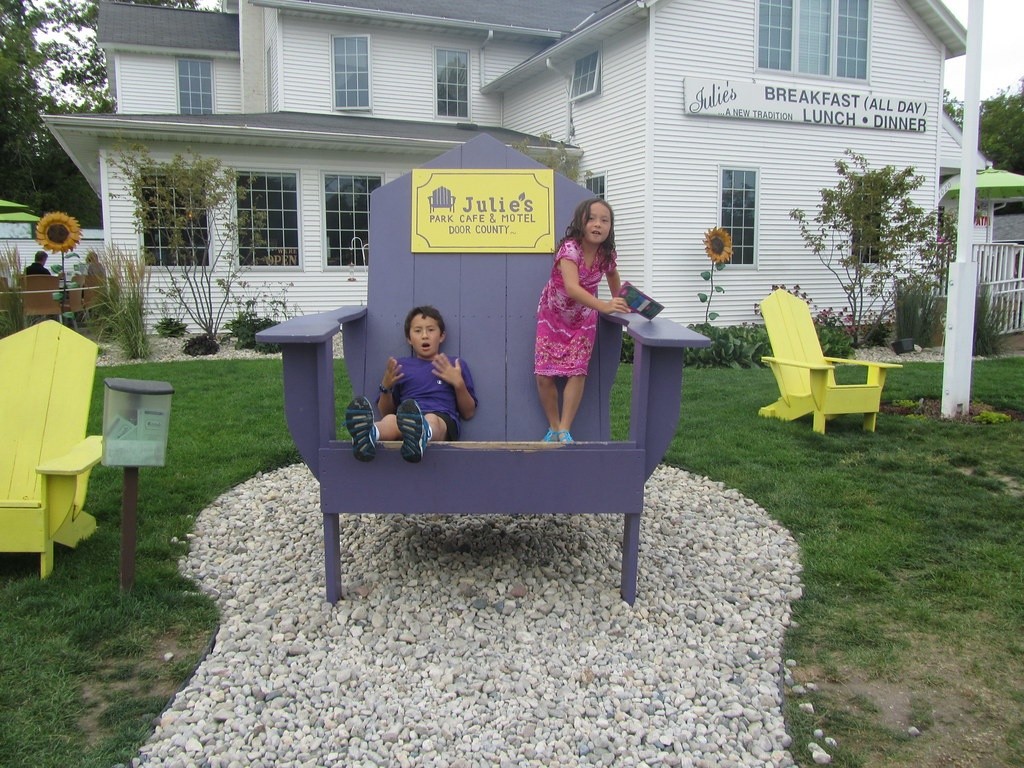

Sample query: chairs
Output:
[[84, 264, 106, 322], [245, 127, 715, 607], [0, 316, 103, 586], [17, 273, 65, 326], [62, 275, 87, 332], [748, 284, 905, 439], [0, 275, 13, 327]]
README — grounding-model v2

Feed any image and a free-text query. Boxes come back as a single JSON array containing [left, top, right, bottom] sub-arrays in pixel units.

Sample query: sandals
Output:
[[541, 428, 558, 441], [558, 430, 575, 444]]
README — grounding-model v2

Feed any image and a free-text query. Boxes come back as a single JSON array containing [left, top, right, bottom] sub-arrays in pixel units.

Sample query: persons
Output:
[[86, 253, 104, 275], [534, 199, 631, 441], [23, 251, 54, 325], [346, 306, 478, 463]]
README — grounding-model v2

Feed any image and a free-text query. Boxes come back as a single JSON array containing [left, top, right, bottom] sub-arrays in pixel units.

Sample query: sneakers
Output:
[[396, 399, 431, 463], [342, 396, 377, 462]]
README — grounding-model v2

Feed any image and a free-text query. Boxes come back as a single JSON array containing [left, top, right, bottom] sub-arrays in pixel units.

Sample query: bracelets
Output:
[[379, 384, 393, 393]]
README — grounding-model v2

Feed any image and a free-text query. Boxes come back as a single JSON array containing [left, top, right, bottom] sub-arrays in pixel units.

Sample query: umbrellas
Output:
[[0, 200, 41, 222], [941, 166, 1024, 243]]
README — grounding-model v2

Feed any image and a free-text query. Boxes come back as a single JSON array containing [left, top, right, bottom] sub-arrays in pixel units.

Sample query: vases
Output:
[[891, 338, 915, 356]]
